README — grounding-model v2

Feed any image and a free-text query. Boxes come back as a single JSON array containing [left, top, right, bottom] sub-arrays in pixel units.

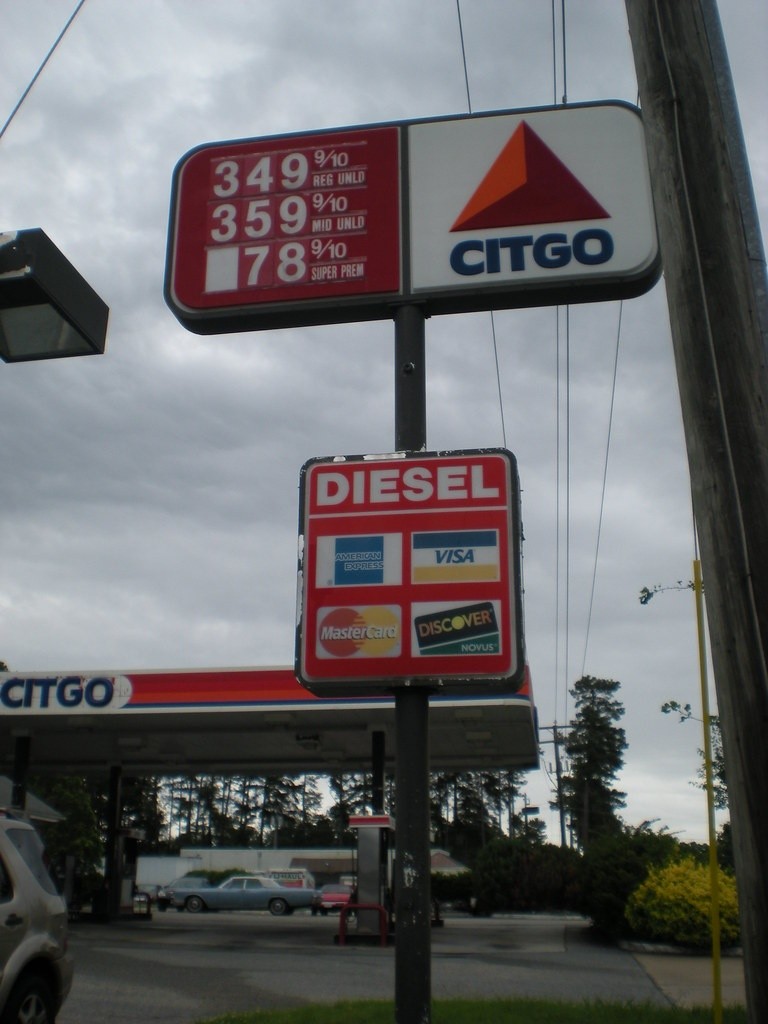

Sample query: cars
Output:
[[171, 875, 324, 916], [318, 882, 354, 915], [157, 878, 211, 913], [135, 882, 166, 905]]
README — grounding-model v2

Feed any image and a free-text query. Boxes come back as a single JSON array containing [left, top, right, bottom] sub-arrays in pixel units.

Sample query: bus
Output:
[[269, 868, 315, 889]]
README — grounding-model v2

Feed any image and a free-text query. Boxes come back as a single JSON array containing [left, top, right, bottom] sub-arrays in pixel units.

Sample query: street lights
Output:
[[521, 791, 541, 912]]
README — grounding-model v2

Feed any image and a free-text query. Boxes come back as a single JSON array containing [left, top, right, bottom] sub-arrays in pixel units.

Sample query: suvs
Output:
[[0, 808, 73, 1024]]
